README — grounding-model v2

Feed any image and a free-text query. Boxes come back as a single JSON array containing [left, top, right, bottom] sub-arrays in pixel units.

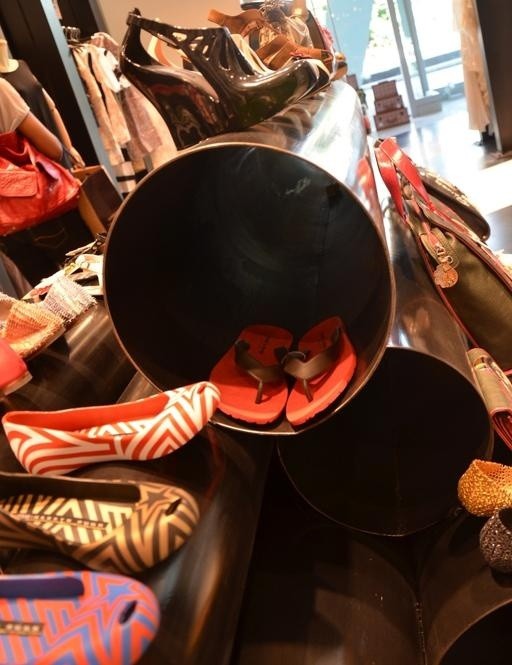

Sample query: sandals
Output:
[[49, 230, 107, 301]]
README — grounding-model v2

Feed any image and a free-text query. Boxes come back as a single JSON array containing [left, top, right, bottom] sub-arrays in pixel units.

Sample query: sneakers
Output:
[[457, 459, 512, 513], [478, 509, 510, 574]]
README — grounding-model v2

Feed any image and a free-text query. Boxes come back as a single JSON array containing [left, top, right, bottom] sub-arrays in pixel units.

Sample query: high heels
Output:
[[118, 7, 351, 149]]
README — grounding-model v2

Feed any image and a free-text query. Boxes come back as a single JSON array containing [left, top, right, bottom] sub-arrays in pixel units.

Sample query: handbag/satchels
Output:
[[0, 126, 83, 244], [373, 127, 512, 376], [463, 343, 512, 450]]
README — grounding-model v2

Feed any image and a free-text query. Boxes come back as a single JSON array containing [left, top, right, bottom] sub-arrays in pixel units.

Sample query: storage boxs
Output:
[[346, 70, 413, 134]]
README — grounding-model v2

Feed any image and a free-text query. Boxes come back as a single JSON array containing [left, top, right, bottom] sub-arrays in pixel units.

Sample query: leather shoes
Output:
[[0, 290, 68, 356], [1, 467, 200, 574], [0, 339, 32, 397], [5, 380, 221, 477]]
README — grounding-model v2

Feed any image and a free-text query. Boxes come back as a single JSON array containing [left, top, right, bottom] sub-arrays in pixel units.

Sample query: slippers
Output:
[[278, 313, 357, 426], [209, 321, 295, 427]]
